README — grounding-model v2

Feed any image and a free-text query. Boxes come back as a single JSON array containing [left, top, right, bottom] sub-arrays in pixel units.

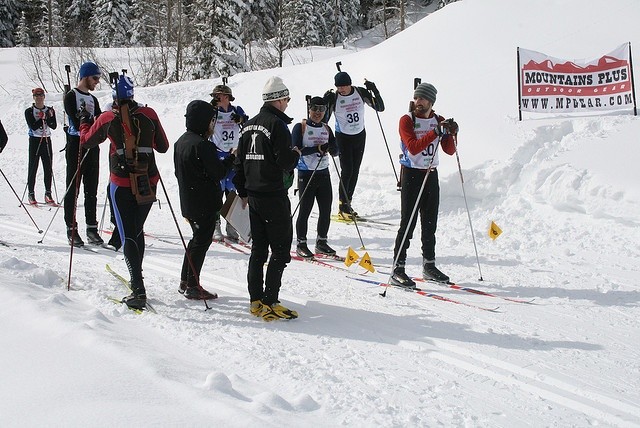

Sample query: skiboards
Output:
[[102, 227, 182, 248], [311, 211, 393, 231], [212, 234, 252, 255], [345, 270, 536, 311], [22, 200, 65, 211], [290, 250, 391, 275], [105, 263, 158, 315], [66, 236, 124, 254]]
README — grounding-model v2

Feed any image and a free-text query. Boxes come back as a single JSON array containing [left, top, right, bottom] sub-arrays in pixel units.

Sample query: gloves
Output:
[[329, 137, 339, 153], [210, 93, 221, 106], [325, 90, 336, 102], [434, 118, 452, 135], [80, 113, 95, 125], [231, 113, 248, 122], [451, 118, 459, 135], [76, 101, 91, 118], [364, 80, 378, 94], [319, 142, 331, 150]]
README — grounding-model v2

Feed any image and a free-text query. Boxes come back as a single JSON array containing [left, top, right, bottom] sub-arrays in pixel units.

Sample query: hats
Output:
[[335, 71, 351, 86], [209, 85, 235, 101], [262, 76, 289, 102], [111, 75, 134, 97], [308, 97, 326, 109], [32, 88, 44, 94], [79, 62, 101, 78], [414, 82, 437, 104]]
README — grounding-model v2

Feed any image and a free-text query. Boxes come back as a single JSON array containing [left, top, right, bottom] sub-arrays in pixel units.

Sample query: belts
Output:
[[401, 165, 436, 172]]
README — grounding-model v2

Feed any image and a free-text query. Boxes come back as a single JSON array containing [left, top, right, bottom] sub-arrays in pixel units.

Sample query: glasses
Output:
[[93, 76, 100, 79], [33, 93, 44, 96], [310, 106, 325, 111]]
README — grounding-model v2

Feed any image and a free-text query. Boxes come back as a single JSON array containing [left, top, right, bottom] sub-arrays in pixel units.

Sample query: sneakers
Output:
[[28, 192, 37, 204], [315, 234, 336, 254], [122, 293, 148, 308], [212, 219, 224, 240], [270, 301, 298, 319], [350, 207, 358, 217], [423, 259, 449, 280], [184, 286, 216, 299], [67, 222, 84, 246], [178, 281, 208, 293], [338, 199, 356, 220], [390, 261, 415, 288], [226, 223, 239, 239], [44, 191, 54, 202], [295, 237, 314, 257], [86, 221, 104, 245], [249, 299, 279, 321]]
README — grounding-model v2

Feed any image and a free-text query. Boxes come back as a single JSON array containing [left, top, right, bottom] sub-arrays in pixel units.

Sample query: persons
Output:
[[325, 72, 385, 220], [174, 100, 235, 300], [232, 76, 302, 320], [24, 88, 58, 205], [64, 62, 107, 247], [207, 85, 250, 241], [391, 83, 459, 290], [293, 96, 337, 259], [80, 76, 170, 308]]
